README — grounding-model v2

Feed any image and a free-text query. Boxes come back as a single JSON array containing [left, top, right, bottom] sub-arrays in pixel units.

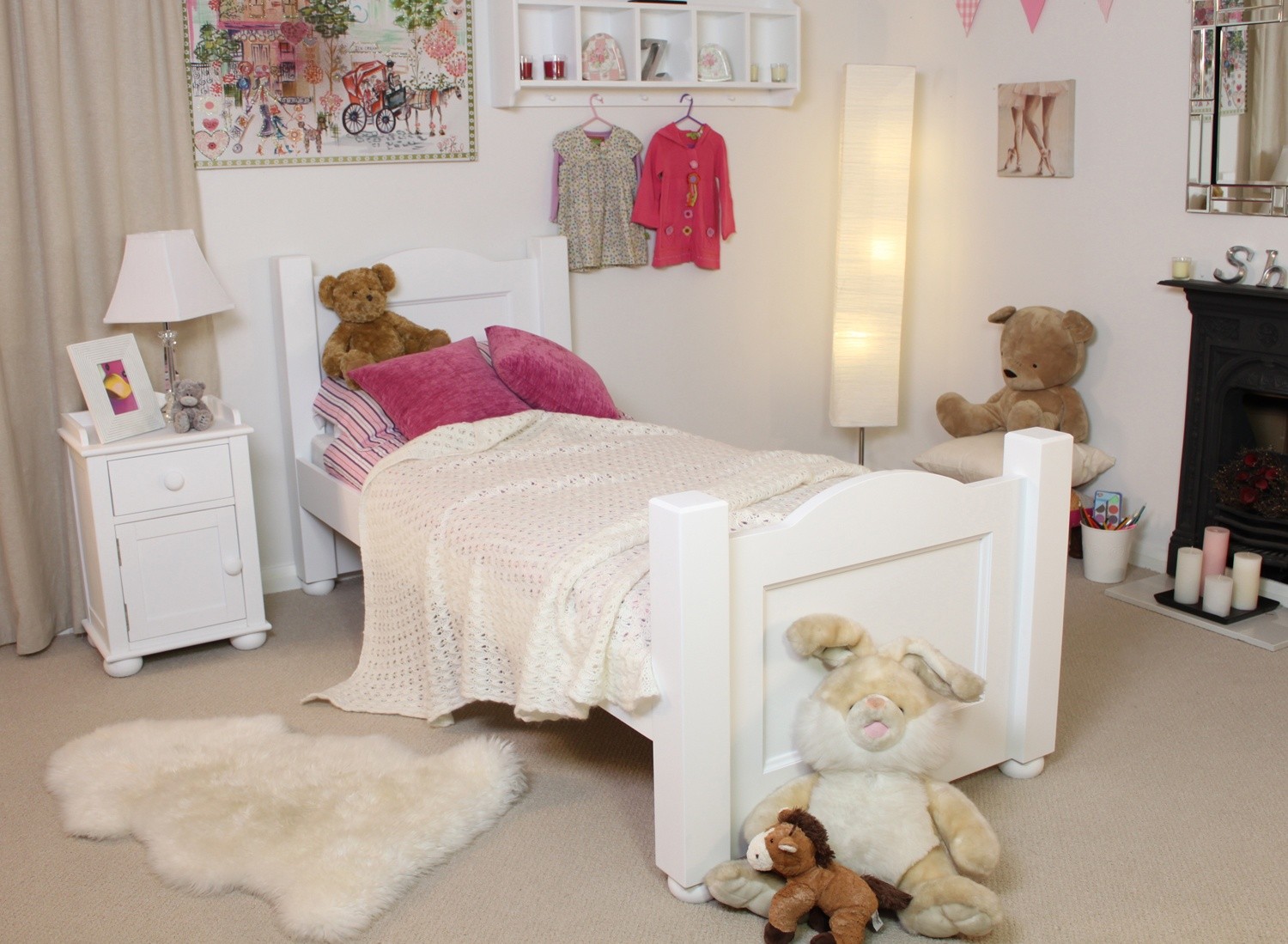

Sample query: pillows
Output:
[[917, 427, 1115, 485], [313, 325, 620, 448]]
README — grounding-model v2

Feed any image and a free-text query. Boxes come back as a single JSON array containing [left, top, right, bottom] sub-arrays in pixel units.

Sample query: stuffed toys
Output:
[[174, 379, 214, 433], [705, 613, 1004, 944], [936, 306, 1093, 444], [319, 264, 451, 391]]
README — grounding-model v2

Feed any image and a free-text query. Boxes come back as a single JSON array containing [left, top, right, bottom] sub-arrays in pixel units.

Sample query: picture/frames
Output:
[[65, 333, 166, 445]]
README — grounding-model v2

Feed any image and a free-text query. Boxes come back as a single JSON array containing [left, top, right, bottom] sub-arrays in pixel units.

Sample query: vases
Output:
[[1207, 504, 1288, 570]]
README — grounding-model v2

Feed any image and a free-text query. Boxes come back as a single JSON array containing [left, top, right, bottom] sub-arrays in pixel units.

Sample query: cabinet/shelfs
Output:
[[1157, 278, 1288, 582], [487, 0, 804, 107]]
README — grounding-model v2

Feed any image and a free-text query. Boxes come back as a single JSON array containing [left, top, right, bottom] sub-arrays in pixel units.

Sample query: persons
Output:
[[1004, 82, 1069, 176]]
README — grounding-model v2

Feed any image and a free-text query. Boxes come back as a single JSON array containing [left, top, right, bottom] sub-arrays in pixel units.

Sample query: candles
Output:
[[1204, 574, 1233, 616], [1232, 551, 1262, 611], [1200, 526, 1229, 596], [1172, 546, 1202, 606]]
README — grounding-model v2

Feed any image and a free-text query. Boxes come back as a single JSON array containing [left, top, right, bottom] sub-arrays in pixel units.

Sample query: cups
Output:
[[520, 55, 533, 80], [543, 54, 566, 80], [1079, 520, 1136, 583], [1170, 256, 1190, 281], [750, 64, 758, 81], [771, 63, 788, 82]]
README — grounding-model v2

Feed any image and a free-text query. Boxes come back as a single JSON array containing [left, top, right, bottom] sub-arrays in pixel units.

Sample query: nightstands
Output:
[[55, 424, 273, 679]]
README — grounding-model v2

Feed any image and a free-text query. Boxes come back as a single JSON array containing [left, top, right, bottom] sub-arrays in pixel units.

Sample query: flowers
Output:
[[1211, 449, 1288, 517]]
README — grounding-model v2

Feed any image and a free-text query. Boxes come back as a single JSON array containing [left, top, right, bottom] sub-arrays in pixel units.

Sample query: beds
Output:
[[276, 233, 1077, 901]]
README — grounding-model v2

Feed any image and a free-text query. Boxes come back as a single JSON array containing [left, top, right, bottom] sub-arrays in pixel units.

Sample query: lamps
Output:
[[104, 229, 236, 425], [832, 66, 917, 468]]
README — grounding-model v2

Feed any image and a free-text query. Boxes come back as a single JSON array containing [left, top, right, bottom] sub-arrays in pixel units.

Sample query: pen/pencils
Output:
[[1078, 502, 1102, 529], [1100, 500, 1147, 530]]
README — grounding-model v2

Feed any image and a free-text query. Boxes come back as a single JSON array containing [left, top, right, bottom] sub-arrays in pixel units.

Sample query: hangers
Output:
[[674, 93, 704, 126], [580, 94, 617, 129]]
